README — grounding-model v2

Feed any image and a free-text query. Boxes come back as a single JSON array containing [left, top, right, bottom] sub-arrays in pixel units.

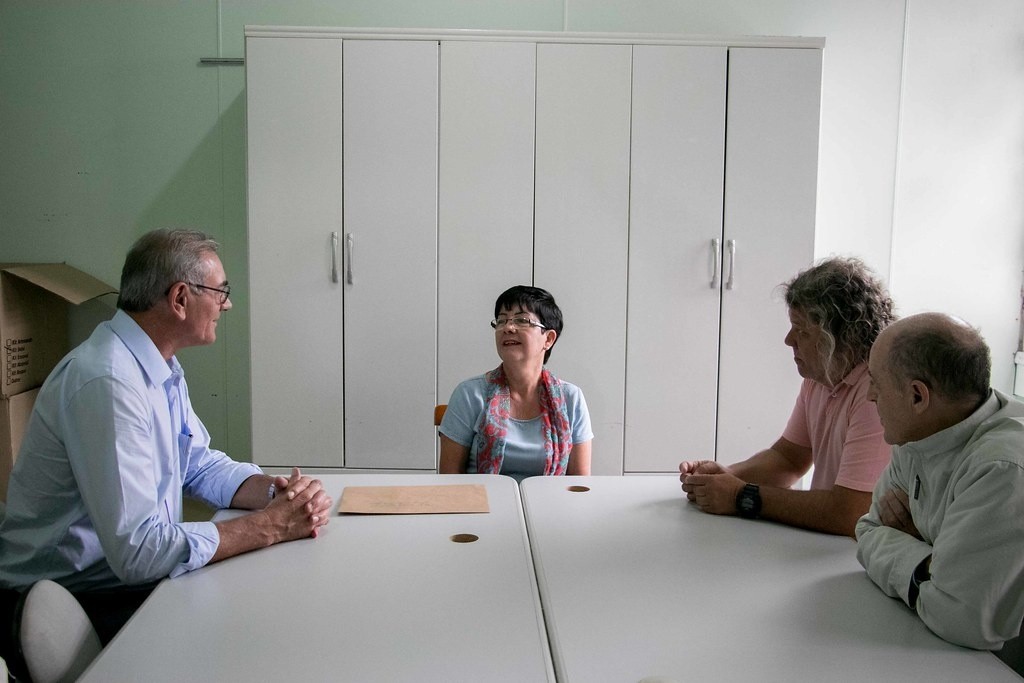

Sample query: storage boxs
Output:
[[0, 262, 119, 400], [0, 386, 42, 507]]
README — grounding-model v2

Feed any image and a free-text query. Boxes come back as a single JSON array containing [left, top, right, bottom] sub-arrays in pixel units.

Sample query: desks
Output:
[[73, 474, 557, 683], [519, 476, 1024, 683]]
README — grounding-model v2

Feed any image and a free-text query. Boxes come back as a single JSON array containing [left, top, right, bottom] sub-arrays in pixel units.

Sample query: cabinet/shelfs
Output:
[[437, 27, 632, 476], [244, 24, 439, 474], [623, 32, 826, 476]]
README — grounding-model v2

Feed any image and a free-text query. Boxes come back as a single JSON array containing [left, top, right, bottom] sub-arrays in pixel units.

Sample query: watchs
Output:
[[737, 483, 762, 519]]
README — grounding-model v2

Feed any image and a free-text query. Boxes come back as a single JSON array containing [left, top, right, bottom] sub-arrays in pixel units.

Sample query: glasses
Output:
[[490, 318, 546, 330], [164, 280, 231, 304]]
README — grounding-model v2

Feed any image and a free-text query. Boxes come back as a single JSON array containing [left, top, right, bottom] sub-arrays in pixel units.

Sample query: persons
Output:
[[438, 285, 595, 484], [0, 226, 332, 683], [855, 311, 1024, 679], [680, 259, 895, 536]]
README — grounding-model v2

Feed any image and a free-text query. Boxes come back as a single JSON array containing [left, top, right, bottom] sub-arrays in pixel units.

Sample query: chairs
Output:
[[11, 577, 105, 682]]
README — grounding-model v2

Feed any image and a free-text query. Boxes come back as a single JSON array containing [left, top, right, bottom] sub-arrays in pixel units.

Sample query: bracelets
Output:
[[268, 483, 275, 499]]
